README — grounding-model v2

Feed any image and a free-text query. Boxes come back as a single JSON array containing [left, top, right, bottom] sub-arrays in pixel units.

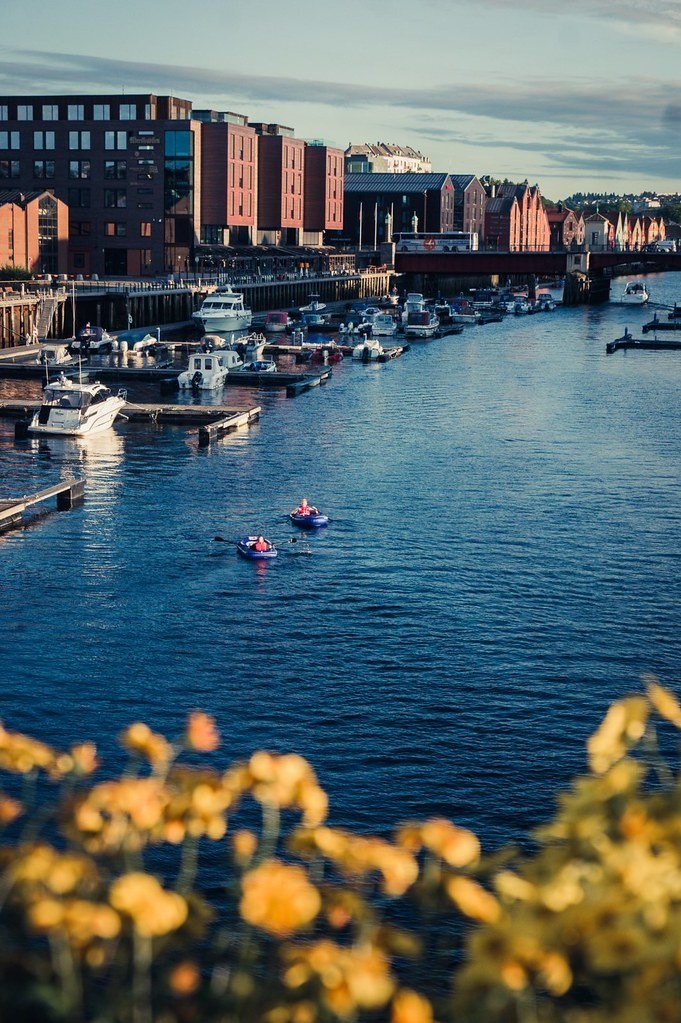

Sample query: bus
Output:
[[391, 231, 479, 252]]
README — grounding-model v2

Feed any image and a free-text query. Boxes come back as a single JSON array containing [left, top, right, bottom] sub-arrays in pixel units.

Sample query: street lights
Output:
[[195, 257, 199, 287]]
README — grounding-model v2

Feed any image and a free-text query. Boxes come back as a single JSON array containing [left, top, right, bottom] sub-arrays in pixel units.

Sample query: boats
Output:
[[190, 285, 255, 335], [158, 353, 229, 395], [237, 536, 278, 559], [71, 323, 113, 355], [289, 506, 332, 529], [620, 280, 651, 304], [15, 354, 129, 440], [82, 283, 563, 375], [35, 345, 74, 366]]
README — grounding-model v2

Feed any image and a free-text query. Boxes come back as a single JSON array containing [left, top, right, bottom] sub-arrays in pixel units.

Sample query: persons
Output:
[[248, 535, 273, 552], [291, 499, 318, 516], [610, 238, 681, 252]]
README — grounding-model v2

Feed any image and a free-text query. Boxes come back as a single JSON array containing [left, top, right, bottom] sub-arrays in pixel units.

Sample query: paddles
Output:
[[274, 537, 298, 546], [214, 535, 246, 547], [285, 509, 301, 523], [317, 509, 333, 521]]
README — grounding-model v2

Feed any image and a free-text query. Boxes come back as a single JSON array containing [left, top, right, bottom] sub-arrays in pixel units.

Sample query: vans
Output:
[[655, 240, 677, 251]]
